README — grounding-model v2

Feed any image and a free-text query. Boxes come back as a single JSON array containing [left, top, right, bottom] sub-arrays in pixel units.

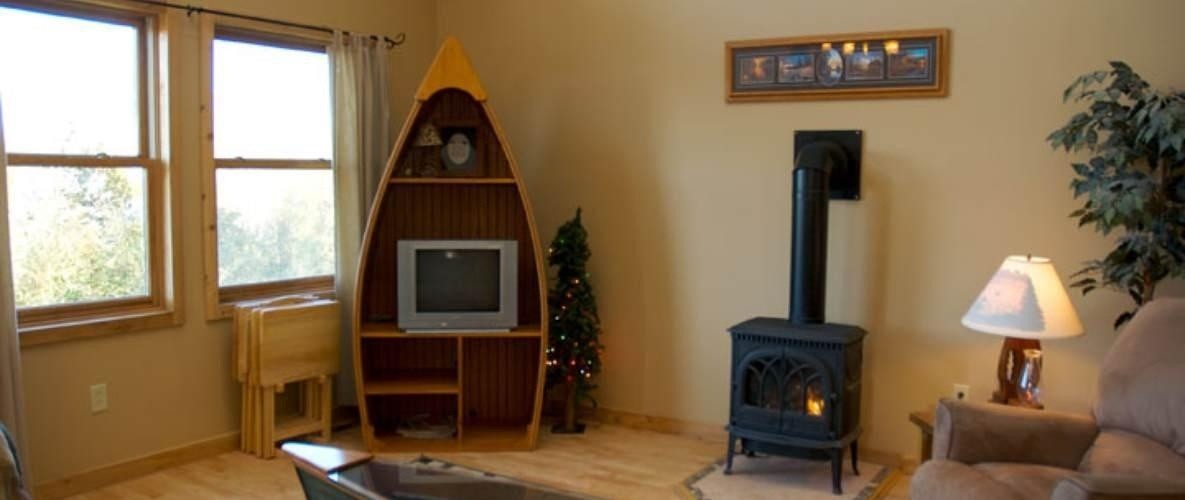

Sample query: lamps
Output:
[[961, 250, 1087, 402]]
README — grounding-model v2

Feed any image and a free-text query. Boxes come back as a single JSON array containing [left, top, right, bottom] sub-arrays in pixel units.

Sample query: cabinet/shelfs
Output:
[[352, 33, 551, 455]]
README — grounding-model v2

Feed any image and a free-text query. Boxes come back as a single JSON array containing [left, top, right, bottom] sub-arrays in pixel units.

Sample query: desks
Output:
[[276, 435, 594, 500], [909, 408, 937, 465]]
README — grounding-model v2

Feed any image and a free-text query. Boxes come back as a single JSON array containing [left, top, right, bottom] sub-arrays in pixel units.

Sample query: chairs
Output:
[[913, 295, 1185, 499]]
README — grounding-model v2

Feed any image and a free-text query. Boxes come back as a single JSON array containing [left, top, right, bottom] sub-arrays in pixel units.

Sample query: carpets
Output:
[[682, 436, 890, 500]]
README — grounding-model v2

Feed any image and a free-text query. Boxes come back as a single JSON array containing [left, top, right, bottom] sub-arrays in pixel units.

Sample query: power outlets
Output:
[[91, 382, 107, 413], [954, 383, 970, 403]]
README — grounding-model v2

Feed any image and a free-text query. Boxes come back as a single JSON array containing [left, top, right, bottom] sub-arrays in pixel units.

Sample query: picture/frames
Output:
[[723, 26, 951, 104]]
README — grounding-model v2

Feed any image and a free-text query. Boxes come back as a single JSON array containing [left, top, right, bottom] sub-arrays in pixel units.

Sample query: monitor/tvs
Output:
[[396, 239, 519, 334]]
